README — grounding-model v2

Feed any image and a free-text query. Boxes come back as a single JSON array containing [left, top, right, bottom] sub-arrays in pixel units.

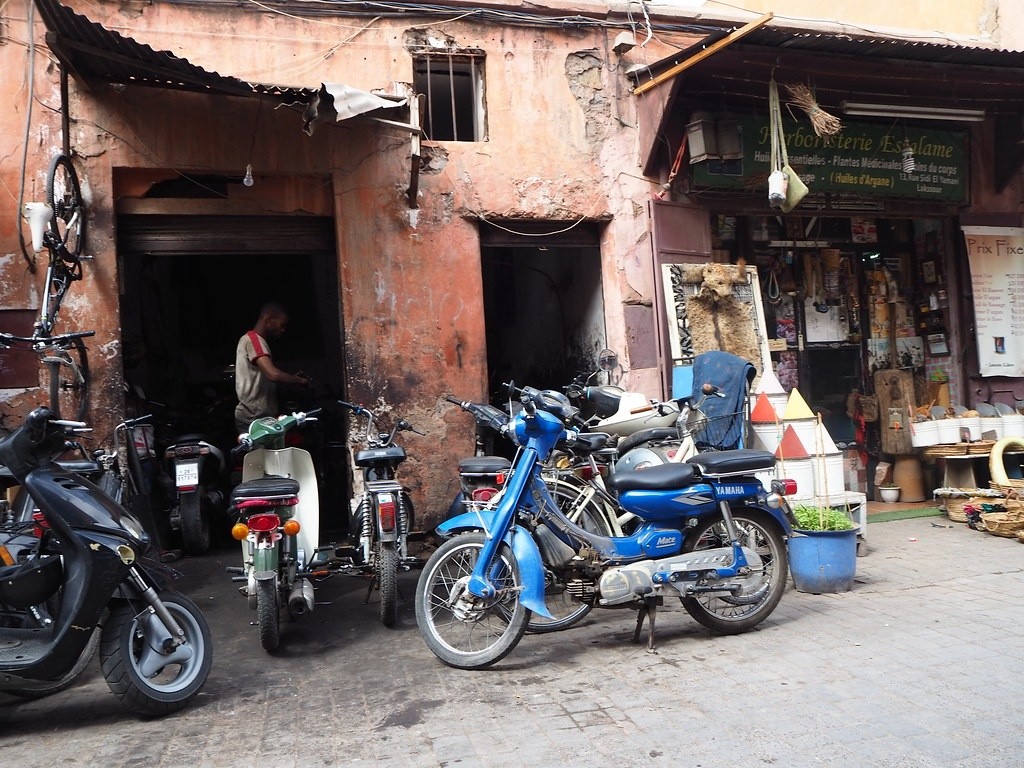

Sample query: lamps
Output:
[[611, 31, 637, 55], [840, 100, 986, 122]]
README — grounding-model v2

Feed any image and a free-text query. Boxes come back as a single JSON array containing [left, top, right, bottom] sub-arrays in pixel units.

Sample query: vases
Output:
[[877, 482, 901, 503]]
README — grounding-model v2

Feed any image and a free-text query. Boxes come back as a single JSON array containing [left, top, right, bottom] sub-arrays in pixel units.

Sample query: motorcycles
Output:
[[0, 403, 214, 719], [133, 363, 236, 553], [330, 399, 426, 626], [414, 347, 797, 668], [225, 408, 333, 650]]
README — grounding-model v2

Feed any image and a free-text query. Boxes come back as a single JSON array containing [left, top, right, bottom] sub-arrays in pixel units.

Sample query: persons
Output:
[[234, 302, 312, 483]]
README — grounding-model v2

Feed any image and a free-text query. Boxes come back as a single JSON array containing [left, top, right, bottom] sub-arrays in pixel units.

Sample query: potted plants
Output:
[[787, 504, 862, 594]]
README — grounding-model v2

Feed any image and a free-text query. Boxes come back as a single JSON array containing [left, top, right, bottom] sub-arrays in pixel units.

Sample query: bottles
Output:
[[930, 293, 937, 311]]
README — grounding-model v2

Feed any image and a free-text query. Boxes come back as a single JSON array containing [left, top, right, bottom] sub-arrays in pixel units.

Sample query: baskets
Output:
[[933, 479, 1024, 542], [685, 412, 745, 453]]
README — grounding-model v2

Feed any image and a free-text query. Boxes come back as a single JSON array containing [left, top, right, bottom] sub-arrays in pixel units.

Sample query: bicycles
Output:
[[0, 154, 95, 427]]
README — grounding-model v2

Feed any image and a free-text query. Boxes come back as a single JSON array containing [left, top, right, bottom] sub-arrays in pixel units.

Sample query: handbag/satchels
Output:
[[767, 79, 810, 215], [846, 389, 879, 422]]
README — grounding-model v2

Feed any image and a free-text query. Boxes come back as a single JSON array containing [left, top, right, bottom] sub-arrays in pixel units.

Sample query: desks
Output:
[[935, 450, 1024, 512]]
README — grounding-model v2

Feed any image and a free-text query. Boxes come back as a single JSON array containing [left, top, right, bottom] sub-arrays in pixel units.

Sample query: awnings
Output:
[[626, 12, 1024, 176]]
